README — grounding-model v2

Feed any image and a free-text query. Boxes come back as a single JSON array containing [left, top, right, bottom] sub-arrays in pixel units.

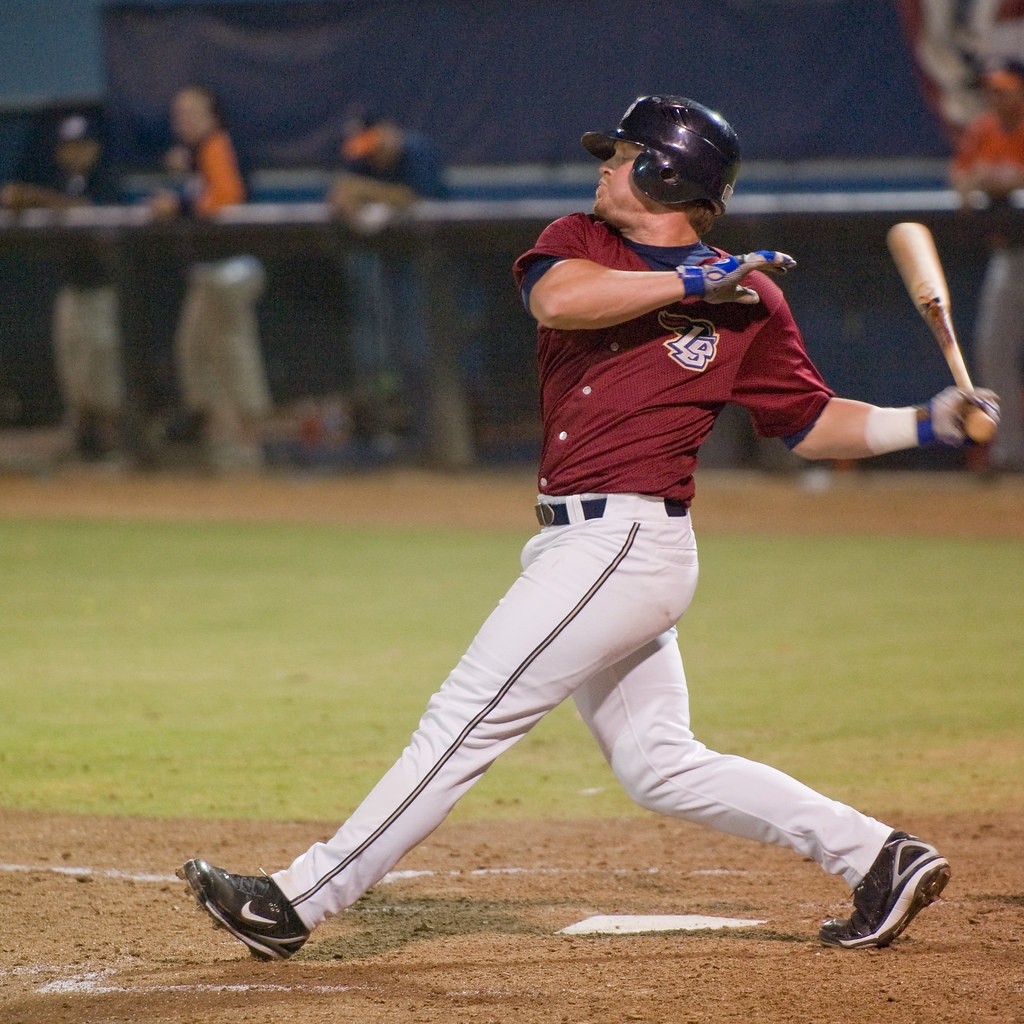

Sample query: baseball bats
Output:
[[886, 222, 999, 442]]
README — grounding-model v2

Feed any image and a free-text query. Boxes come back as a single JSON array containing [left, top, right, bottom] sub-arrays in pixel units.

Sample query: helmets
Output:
[[581, 93, 744, 218]]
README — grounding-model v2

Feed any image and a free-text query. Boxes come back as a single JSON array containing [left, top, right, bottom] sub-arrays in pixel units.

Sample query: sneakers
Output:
[[177, 858, 311, 962], [818, 832, 952, 949]]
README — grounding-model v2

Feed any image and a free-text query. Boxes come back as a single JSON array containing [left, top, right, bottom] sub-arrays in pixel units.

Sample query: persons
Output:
[[953, 54, 1023, 476], [178, 95, 1002, 965], [1, 90, 474, 478]]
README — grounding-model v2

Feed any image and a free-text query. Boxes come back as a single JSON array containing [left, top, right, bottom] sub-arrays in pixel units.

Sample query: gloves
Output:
[[914, 383, 1003, 452], [676, 249, 798, 305]]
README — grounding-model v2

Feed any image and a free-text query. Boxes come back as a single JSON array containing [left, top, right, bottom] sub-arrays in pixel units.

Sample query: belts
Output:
[[535, 499, 687, 525]]
